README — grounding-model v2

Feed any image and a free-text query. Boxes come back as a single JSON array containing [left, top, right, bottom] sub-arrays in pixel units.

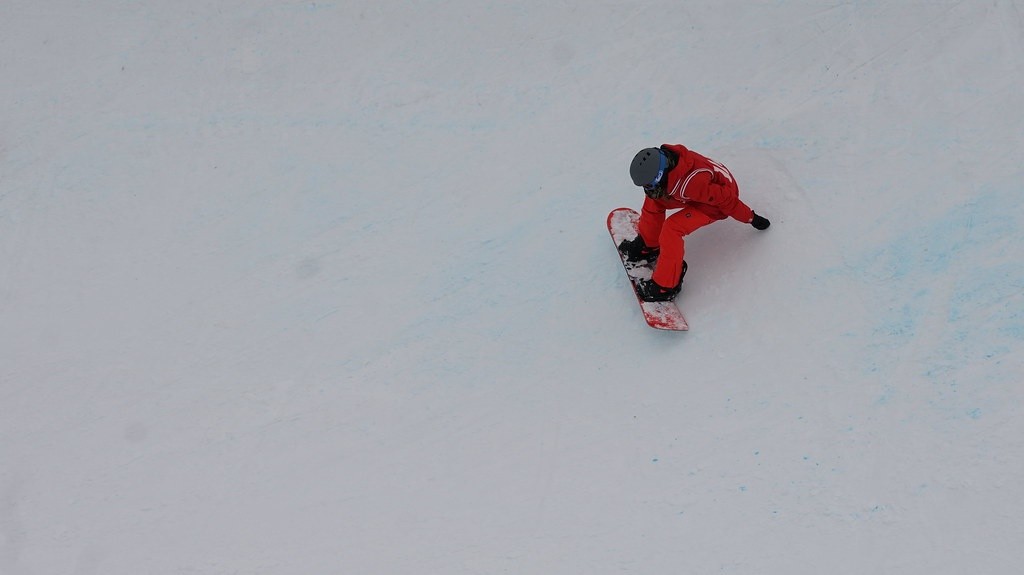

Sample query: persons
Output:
[[616, 143, 771, 303]]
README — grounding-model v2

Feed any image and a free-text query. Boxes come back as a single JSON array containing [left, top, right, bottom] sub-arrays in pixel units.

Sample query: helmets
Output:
[[630, 147, 661, 187]]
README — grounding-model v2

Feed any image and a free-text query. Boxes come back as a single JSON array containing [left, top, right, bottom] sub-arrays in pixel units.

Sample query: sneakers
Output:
[[629, 234, 660, 257], [645, 279, 675, 296]]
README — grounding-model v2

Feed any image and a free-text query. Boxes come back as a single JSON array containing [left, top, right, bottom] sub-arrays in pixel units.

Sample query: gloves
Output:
[[751, 209, 770, 231]]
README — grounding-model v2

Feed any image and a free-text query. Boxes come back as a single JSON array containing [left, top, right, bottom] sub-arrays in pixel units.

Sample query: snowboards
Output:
[[607, 208, 690, 331]]
